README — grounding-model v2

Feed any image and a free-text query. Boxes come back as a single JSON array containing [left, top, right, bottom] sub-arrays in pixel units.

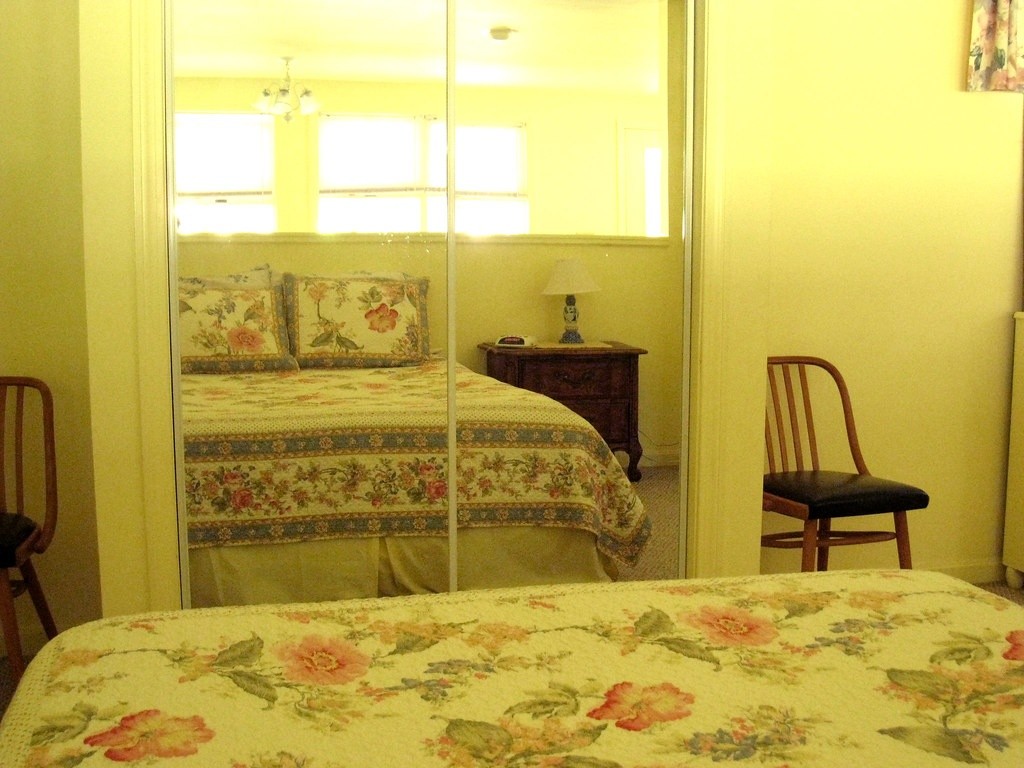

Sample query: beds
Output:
[[0, 571, 1024, 768], [179, 261, 651, 608]]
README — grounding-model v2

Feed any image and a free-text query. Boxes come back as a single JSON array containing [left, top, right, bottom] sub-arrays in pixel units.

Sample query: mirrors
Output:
[[163, 0, 700, 610]]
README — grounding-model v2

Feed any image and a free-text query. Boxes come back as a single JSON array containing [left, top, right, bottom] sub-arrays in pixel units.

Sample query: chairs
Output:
[[0, 377, 58, 693], [761, 356, 929, 572]]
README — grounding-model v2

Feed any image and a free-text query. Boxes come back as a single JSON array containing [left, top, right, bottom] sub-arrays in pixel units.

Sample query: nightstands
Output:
[[477, 341, 649, 482]]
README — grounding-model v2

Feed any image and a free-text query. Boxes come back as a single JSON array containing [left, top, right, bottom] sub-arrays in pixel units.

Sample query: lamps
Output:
[[543, 270, 602, 343]]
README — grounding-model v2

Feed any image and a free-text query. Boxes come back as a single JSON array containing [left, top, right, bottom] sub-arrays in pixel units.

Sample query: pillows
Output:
[[283, 271, 432, 368], [178, 264, 301, 373]]
